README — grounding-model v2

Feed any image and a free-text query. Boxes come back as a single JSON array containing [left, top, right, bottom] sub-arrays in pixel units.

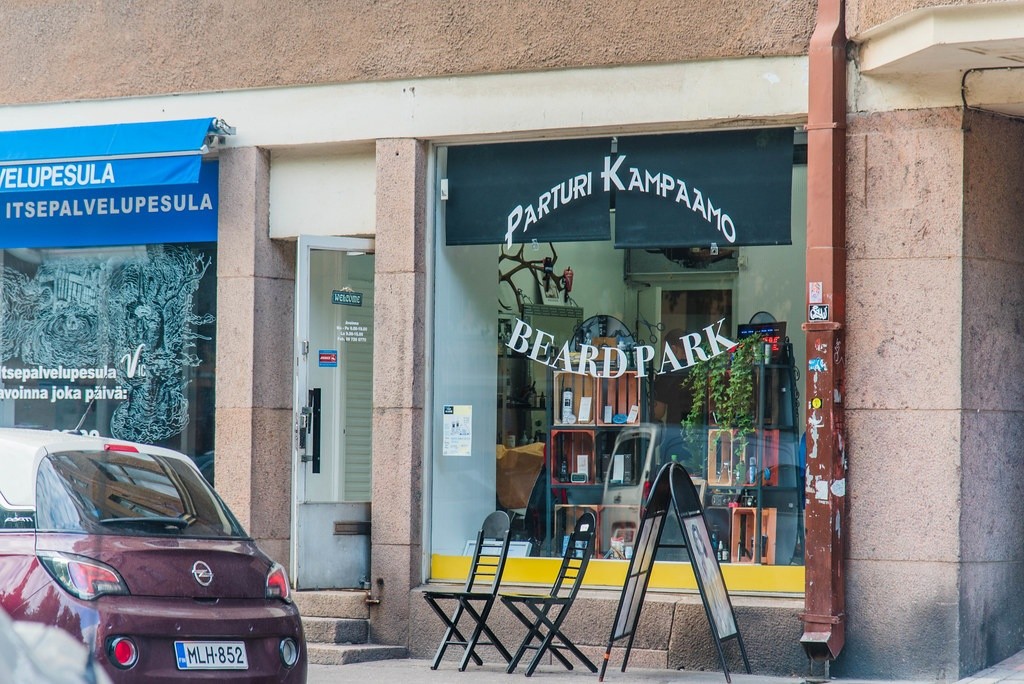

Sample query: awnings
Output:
[[0, 118, 217, 193]]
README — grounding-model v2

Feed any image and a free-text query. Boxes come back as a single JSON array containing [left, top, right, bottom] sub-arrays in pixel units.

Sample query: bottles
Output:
[[562, 456, 567, 481], [718, 541, 728, 561], [562, 388, 573, 424], [712, 524, 719, 556], [540, 392, 545, 408], [750, 457, 755, 483], [721, 463, 728, 482]]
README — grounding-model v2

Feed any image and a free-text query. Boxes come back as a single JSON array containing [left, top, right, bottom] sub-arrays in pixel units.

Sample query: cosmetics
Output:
[[604, 404, 639, 423], [561, 454, 590, 483], [563, 387, 592, 423], [602, 453, 631, 481], [508, 430, 539, 448], [528, 390, 545, 408]]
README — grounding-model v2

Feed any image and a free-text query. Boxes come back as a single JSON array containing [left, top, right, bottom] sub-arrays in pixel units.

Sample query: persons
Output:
[[650, 329, 697, 471], [693, 525, 720, 609]]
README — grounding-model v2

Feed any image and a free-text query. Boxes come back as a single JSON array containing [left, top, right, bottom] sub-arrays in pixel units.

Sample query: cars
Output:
[[0, 428, 308, 684]]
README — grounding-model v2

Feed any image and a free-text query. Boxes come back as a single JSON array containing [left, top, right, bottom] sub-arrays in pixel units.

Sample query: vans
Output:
[[598, 422, 803, 566]]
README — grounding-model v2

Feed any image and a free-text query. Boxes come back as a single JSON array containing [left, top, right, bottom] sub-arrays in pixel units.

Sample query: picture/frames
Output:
[[578, 397, 593, 423], [535, 263, 565, 305], [577, 454, 591, 483]]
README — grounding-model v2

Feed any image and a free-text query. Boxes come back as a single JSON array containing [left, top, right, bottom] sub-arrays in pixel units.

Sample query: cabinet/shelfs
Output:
[[547, 346, 804, 564]]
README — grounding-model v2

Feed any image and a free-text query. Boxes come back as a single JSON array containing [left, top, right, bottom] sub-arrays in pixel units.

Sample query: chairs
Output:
[[421, 512, 517, 673], [501, 511, 597, 676]]
